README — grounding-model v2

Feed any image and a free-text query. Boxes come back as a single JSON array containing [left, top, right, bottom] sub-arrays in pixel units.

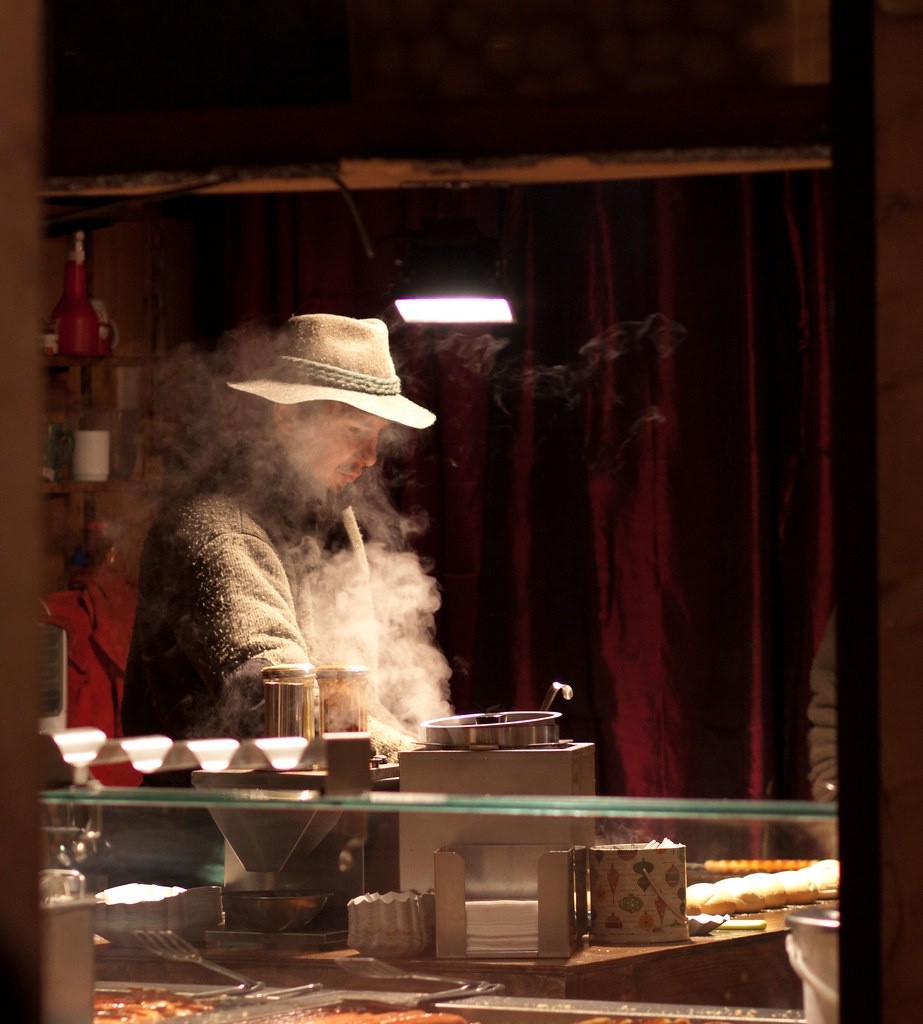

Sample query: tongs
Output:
[[128, 930, 263, 1001], [336, 958, 504, 1008]]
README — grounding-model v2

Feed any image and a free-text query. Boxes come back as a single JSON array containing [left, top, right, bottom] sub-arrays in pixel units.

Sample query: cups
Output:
[[91, 299, 119, 357], [72, 430, 109, 481], [43, 423, 74, 481]]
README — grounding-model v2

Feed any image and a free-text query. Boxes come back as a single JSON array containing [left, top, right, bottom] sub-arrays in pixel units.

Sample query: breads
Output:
[[684, 858, 841, 917]]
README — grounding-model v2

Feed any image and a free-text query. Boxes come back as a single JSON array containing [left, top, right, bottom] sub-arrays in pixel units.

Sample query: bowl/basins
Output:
[[226, 891, 333, 930], [422, 712, 562, 729]]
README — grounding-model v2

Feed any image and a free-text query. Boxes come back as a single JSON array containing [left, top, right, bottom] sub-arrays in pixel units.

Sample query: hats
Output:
[[226, 313, 438, 429]]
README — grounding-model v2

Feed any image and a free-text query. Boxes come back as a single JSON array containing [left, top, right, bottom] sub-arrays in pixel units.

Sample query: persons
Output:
[[122, 314, 390, 886]]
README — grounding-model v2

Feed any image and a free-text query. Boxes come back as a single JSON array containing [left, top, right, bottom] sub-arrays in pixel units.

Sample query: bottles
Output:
[[47, 231, 98, 356]]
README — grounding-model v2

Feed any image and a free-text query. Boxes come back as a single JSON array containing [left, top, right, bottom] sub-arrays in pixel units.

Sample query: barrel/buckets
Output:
[[785, 909, 841, 1024], [589, 844, 689, 943]]
